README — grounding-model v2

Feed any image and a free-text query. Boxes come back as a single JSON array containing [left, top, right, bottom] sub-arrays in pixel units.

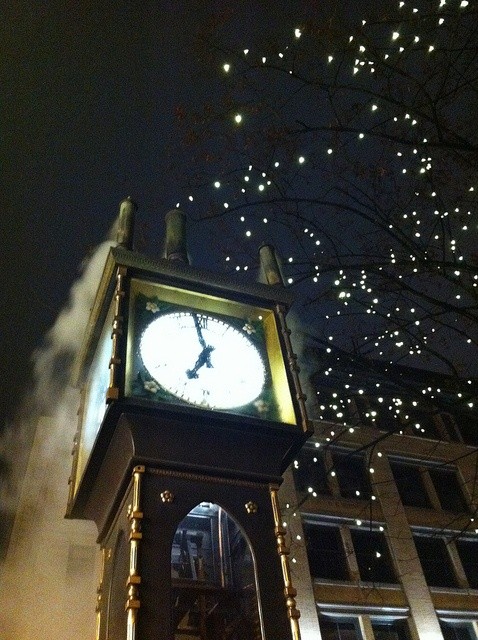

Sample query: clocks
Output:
[[126, 277, 296, 425]]
